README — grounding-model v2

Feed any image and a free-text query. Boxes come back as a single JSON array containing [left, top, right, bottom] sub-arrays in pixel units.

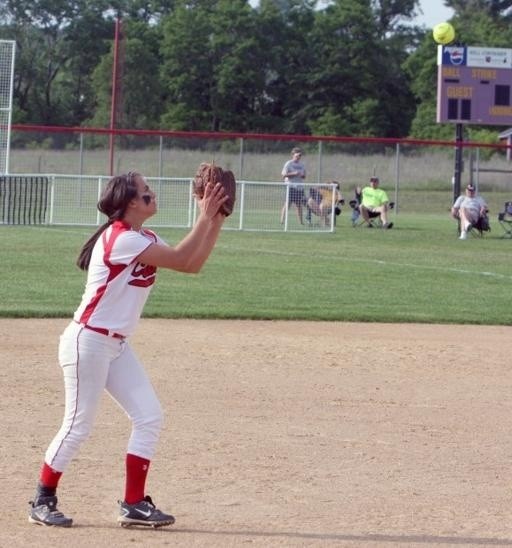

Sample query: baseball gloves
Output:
[[193, 161, 236, 216]]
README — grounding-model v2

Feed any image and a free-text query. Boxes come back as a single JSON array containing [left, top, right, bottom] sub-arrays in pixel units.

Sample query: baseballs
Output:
[[434, 22, 456, 42]]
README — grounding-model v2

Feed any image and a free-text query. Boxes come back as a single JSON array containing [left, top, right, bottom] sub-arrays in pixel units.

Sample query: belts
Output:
[[85, 325, 125, 338]]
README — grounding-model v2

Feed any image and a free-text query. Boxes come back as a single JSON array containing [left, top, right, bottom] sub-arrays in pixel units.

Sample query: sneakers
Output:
[[28, 496, 72, 526], [118, 496, 174, 525], [459, 222, 471, 240], [384, 222, 392, 228]]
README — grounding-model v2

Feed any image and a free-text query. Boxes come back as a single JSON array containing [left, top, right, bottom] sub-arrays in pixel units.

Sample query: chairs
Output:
[[458, 201, 512, 240], [301, 181, 398, 229]]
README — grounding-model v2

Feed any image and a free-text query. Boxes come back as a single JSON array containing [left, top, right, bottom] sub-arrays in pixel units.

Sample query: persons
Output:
[[307, 180, 339, 229], [27, 171, 225, 530], [356, 177, 393, 230], [452, 183, 488, 240], [280, 146, 306, 225]]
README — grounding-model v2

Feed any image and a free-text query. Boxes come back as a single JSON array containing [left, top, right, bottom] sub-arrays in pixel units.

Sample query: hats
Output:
[[466, 184, 475, 191], [370, 178, 378, 182]]
